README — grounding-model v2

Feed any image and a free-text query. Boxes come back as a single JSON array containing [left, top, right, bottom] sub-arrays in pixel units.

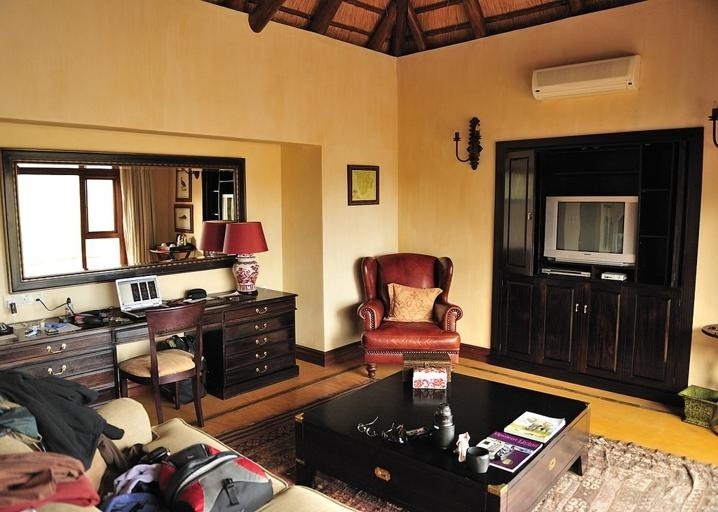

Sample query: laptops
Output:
[[116, 274, 170, 318]]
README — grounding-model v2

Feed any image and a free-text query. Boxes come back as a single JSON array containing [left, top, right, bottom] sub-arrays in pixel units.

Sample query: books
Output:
[[475, 410, 566, 473]]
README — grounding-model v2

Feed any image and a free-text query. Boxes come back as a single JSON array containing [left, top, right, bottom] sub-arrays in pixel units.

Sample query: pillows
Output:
[[384, 283, 444, 323]]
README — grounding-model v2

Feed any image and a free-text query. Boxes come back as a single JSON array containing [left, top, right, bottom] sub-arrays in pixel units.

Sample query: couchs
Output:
[[357, 253, 463, 378], [0, 397, 360, 511]]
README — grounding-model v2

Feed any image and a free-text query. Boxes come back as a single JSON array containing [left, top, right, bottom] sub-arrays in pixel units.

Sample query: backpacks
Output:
[[156, 335, 209, 404], [159, 443, 274, 512]]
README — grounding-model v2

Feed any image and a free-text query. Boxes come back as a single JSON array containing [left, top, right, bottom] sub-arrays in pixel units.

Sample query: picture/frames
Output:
[[347, 165, 379, 206], [175, 168, 192, 202], [174, 204, 193, 233]]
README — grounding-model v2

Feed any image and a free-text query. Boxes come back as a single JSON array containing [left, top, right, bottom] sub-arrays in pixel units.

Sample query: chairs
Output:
[[119, 300, 210, 429]]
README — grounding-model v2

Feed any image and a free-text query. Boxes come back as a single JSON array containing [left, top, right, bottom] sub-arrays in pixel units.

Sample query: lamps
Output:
[[454, 117, 482, 172], [199, 220, 237, 257], [223, 222, 268, 296]]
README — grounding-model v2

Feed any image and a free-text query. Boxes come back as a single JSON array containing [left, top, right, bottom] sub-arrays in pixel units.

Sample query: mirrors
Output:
[[17, 163, 236, 279]]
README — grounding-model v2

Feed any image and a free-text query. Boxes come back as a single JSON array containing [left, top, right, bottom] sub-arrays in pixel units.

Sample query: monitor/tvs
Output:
[[543, 195, 639, 266]]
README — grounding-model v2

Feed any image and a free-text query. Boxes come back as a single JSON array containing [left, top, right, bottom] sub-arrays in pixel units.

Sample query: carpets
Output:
[[215, 379, 718, 512]]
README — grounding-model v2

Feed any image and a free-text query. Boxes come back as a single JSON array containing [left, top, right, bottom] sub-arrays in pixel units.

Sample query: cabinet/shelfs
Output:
[[545, 286, 621, 372], [628, 297, 676, 378], [635, 138, 679, 291], [202, 168, 236, 220], [501, 147, 541, 276], [500, 278, 539, 356], [544, 150, 636, 177]]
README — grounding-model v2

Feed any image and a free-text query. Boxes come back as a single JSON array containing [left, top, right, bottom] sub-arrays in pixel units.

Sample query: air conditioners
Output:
[[531, 54, 638, 101]]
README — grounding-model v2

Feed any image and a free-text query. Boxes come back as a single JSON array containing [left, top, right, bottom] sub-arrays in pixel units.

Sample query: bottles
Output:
[[430, 402, 455, 450]]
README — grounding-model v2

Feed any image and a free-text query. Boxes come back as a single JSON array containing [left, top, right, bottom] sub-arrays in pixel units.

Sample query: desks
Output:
[[701, 324, 718, 339], [149, 243, 197, 261], [0, 287, 299, 400]]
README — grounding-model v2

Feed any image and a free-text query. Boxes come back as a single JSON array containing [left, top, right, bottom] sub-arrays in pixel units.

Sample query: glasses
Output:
[[357, 417, 395, 437]]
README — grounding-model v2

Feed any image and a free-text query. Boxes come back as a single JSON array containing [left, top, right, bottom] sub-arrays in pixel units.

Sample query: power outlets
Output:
[[32, 294, 43, 303], [4, 295, 16, 308]]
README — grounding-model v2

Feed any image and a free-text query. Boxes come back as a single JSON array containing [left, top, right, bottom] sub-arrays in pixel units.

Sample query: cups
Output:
[[465, 446, 490, 477]]
